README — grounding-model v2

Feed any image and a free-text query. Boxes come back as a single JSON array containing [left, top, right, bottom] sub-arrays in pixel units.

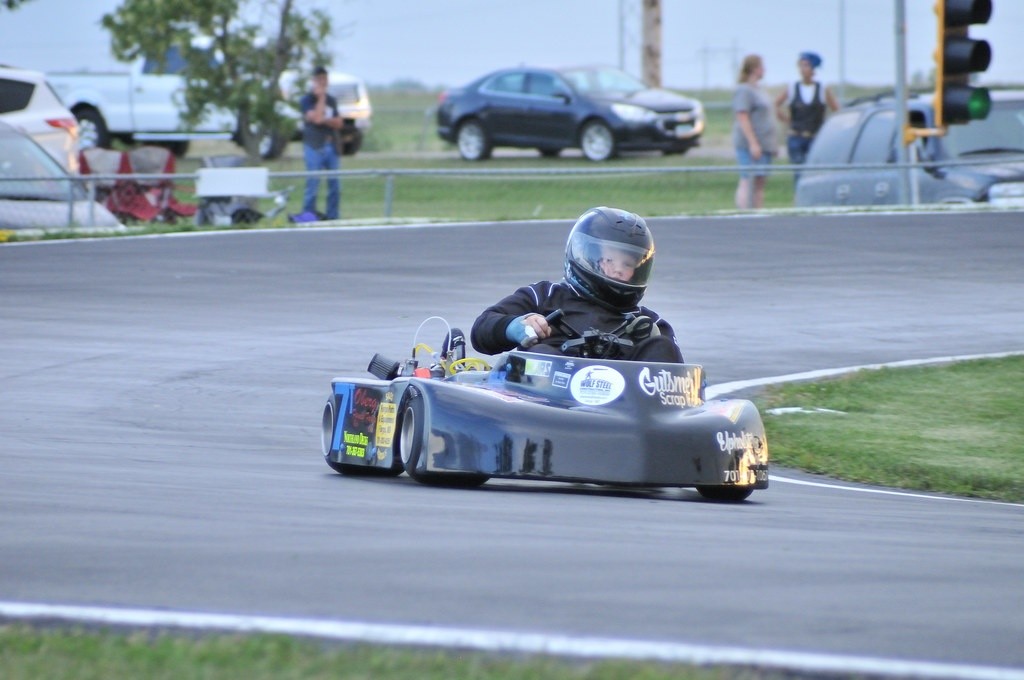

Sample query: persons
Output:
[[470, 206, 685, 365], [300, 65, 344, 220], [773, 51, 840, 186], [732, 54, 779, 208]]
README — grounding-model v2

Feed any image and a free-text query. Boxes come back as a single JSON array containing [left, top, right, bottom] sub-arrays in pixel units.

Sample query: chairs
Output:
[[77, 144, 198, 228]]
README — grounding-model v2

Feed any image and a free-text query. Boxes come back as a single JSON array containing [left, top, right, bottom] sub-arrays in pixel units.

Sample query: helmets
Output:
[[564, 206, 654, 311]]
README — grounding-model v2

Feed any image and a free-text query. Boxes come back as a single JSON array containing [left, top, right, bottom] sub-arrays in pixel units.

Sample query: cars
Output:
[[1, 124, 125, 230], [0, 63, 85, 200], [430, 63, 707, 163]]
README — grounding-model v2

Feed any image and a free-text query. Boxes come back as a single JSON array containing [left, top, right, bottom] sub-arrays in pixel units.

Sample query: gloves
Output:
[[623, 315, 653, 345]]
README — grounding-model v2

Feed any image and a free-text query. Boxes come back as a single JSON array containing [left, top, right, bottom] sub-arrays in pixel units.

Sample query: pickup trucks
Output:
[[43, 38, 372, 160]]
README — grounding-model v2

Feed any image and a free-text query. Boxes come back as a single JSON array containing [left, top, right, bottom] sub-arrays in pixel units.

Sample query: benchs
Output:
[[195, 166, 296, 228]]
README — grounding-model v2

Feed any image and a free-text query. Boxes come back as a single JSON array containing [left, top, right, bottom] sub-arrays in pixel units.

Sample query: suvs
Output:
[[792, 89, 1024, 206]]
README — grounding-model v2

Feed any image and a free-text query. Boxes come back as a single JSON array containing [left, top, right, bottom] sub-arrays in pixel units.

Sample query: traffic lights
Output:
[[931, 0, 997, 129]]
